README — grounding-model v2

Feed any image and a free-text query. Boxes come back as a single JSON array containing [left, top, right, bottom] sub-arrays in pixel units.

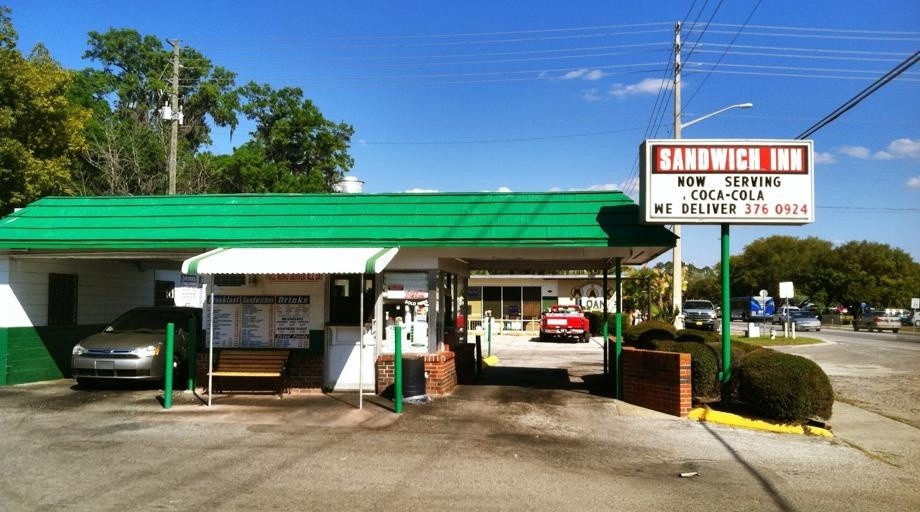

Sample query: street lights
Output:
[[667, 100, 756, 326]]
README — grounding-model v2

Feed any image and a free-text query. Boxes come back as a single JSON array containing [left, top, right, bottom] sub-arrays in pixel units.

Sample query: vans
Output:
[[778, 306, 801, 324]]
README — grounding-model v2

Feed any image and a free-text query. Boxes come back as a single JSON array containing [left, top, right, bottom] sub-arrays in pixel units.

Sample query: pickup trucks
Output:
[[539, 303, 590, 343]]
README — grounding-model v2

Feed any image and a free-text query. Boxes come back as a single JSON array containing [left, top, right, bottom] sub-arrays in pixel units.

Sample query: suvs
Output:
[[682, 298, 717, 330]]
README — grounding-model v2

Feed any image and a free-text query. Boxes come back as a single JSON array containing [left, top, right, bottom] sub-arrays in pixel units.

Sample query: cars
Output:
[[69, 304, 205, 390], [782, 310, 822, 331], [824, 304, 920, 333], [715, 306, 721, 317]]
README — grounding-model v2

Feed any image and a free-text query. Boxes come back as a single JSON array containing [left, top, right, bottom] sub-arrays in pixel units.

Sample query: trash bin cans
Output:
[[402, 357, 425, 397]]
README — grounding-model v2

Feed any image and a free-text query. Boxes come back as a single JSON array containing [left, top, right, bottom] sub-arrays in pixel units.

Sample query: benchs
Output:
[[206, 350, 290, 398]]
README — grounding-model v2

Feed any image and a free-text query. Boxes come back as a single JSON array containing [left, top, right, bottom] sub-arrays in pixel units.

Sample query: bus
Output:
[[731, 296, 775, 321]]
